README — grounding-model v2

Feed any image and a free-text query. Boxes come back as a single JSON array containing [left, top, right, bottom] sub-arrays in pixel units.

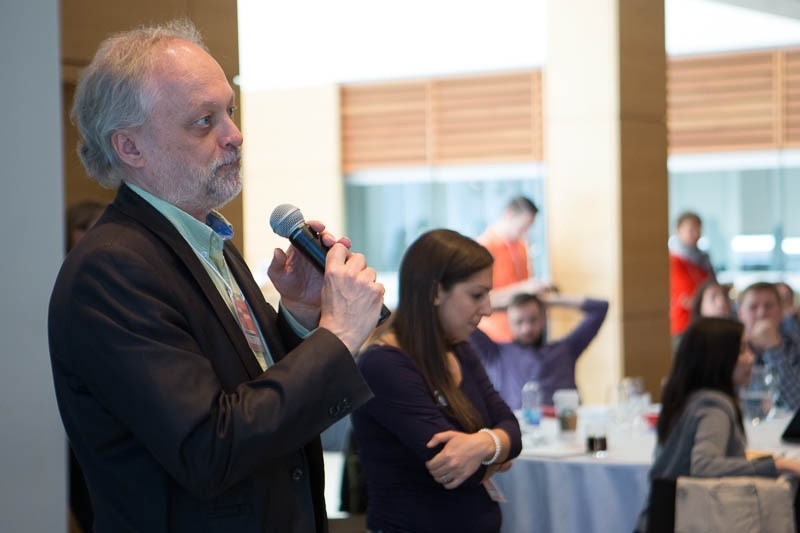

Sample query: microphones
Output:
[[269, 204, 391, 328]]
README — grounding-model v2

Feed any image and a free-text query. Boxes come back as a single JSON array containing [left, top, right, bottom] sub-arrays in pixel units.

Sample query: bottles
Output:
[[521, 376, 541, 426], [587, 432, 609, 457]]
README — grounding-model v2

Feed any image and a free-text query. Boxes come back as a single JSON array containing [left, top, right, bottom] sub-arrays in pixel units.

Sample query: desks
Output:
[[488, 404, 800, 533]]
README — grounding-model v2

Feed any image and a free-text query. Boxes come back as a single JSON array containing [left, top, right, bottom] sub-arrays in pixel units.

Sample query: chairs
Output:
[[647, 475, 800, 533]]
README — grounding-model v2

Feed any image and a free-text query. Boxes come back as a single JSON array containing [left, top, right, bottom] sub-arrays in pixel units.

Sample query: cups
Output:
[[739, 366, 775, 425], [551, 388, 579, 431]]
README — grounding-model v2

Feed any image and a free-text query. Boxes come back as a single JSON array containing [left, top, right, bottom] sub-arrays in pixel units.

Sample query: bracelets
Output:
[[478, 428, 500, 466]]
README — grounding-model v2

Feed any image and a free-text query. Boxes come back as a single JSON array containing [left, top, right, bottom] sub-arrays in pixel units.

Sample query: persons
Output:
[[470, 198, 561, 344], [635, 213, 800, 533], [469, 295, 608, 412], [47, 17, 386, 532], [352, 228, 523, 533]]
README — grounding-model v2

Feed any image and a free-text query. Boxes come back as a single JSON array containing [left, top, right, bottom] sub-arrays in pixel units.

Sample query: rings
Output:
[[443, 476, 450, 481]]
